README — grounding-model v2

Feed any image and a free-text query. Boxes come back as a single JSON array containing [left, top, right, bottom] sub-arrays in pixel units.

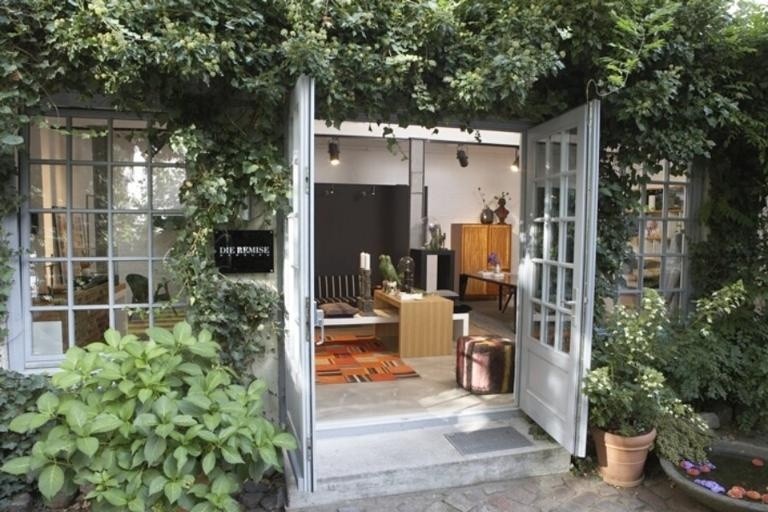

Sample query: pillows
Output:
[[317, 302, 360, 317]]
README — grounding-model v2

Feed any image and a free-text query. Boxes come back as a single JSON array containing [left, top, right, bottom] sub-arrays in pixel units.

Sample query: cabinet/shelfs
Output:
[[451, 224, 512, 300], [32, 281, 125, 355]]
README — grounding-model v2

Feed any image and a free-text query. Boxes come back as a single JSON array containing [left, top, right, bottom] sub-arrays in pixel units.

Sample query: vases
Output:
[[481, 207, 494, 224], [495, 264, 501, 273]]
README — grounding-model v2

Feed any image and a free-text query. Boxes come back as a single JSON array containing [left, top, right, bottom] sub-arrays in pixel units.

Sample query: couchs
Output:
[[314, 274, 386, 327]]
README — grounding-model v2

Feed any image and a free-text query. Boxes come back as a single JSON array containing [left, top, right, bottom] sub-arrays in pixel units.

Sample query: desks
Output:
[[410, 248, 455, 291], [374, 289, 455, 358], [459, 271, 517, 313]]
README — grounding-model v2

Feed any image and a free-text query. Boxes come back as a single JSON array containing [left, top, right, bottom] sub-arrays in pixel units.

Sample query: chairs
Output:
[[126, 274, 177, 321]]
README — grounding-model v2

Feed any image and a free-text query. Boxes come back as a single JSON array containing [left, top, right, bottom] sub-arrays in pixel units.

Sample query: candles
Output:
[[360, 251, 371, 270]]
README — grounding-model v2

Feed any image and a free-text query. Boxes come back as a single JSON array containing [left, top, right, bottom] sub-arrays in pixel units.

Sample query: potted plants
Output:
[[582, 365, 668, 486]]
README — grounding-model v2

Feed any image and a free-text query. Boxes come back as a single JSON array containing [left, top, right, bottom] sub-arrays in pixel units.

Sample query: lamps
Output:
[[328, 137, 341, 164], [456, 144, 469, 167], [511, 148, 519, 172]]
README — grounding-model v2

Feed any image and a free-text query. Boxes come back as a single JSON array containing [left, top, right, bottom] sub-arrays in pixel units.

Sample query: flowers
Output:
[[487, 252, 500, 267]]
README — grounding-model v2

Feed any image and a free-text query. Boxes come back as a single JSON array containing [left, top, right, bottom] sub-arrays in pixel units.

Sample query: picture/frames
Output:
[[51, 206, 87, 285]]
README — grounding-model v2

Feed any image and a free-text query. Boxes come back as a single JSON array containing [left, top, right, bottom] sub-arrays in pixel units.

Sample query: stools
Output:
[[456, 334, 514, 394]]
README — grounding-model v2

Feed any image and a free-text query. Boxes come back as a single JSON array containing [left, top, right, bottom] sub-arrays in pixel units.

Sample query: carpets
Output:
[[315, 334, 421, 385]]
